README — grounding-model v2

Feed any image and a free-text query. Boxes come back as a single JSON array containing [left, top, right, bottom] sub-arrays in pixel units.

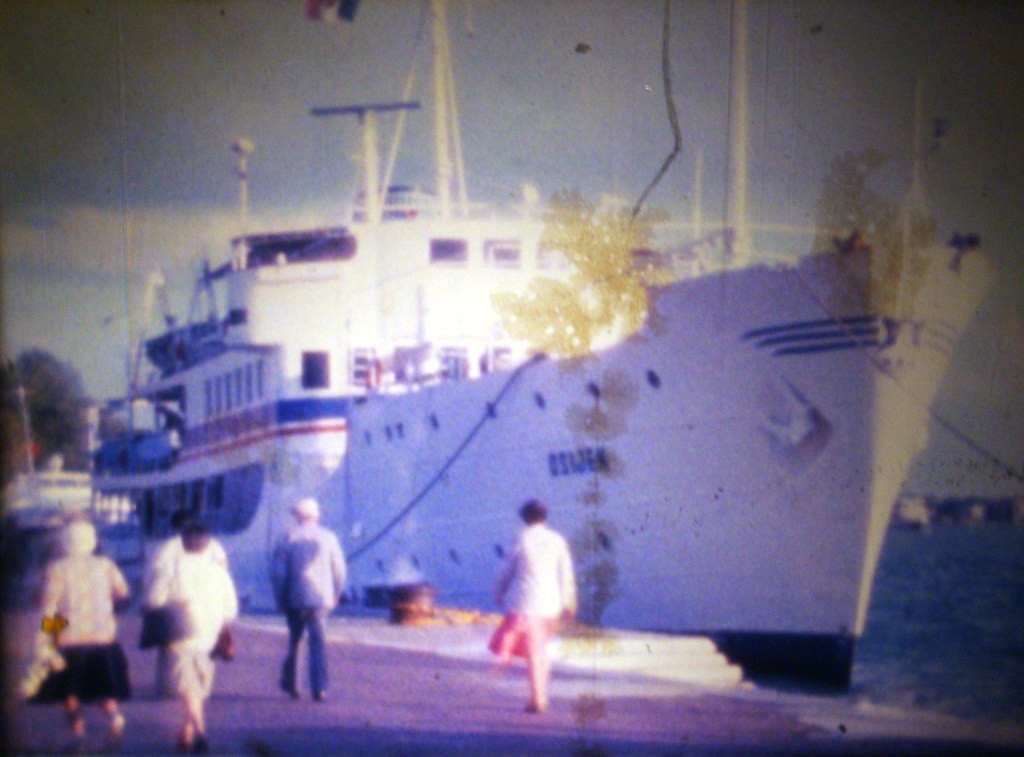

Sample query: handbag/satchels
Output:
[[138, 604, 190, 650]]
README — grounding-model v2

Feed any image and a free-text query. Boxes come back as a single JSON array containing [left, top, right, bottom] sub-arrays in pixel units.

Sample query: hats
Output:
[[62, 522, 96, 552], [292, 497, 321, 519]]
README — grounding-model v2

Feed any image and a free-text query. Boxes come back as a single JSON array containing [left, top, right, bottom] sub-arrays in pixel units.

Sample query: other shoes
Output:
[[315, 691, 326, 702], [288, 687, 300, 699]]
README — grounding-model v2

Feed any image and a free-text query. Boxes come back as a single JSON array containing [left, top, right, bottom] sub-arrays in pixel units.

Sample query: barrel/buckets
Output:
[[393, 584, 436, 622]]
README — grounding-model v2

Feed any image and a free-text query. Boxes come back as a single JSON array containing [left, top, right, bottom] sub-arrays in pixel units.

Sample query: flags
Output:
[[304, 0, 358, 22]]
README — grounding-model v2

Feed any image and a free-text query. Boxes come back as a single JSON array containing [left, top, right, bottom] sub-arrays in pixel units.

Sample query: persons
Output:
[[493, 498, 576, 713], [275, 500, 348, 707], [37, 509, 239, 757]]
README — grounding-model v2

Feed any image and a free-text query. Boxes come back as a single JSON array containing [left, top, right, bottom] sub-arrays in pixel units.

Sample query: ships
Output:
[[91, 2, 989, 702]]
